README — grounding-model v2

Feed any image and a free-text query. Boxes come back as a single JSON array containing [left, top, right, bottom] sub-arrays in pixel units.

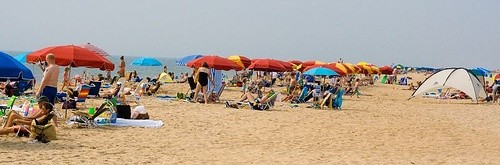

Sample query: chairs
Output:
[[236, 92, 278, 111], [13, 112, 55, 139], [115, 77, 126, 96], [124, 78, 149, 105], [291, 86, 308, 104], [70, 101, 110, 128], [0, 95, 18, 115], [87, 80, 102, 99], [297, 89, 316, 104], [100, 78, 126, 102], [194, 83, 226, 102], [73, 83, 96, 102], [19, 100, 31, 117]]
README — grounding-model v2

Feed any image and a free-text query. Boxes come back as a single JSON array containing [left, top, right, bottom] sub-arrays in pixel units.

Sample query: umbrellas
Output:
[[176, 54, 206, 66], [248, 58, 414, 72], [188, 55, 241, 71], [14, 51, 35, 73], [227, 55, 251, 76], [27, 45, 115, 119], [81, 43, 110, 57], [0, 52, 34, 81]]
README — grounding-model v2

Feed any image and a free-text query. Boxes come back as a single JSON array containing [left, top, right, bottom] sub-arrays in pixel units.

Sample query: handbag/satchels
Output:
[[29, 119, 57, 143]]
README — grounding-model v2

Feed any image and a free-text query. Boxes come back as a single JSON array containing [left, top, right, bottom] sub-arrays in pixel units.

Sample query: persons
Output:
[[61, 67, 71, 90], [99, 83, 121, 98], [486, 80, 500, 102], [36, 54, 59, 105], [4, 95, 49, 127], [191, 62, 216, 105], [225, 70, 414, 117], [115, 55, 195, 120], [0, 102, 53, 136]]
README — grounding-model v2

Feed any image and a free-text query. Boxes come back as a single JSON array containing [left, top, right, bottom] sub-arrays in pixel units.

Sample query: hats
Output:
[[37, 96, 49, 102]]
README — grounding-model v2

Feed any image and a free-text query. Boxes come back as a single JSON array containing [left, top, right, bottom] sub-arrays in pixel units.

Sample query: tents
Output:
[[410, 68, 487, 103]]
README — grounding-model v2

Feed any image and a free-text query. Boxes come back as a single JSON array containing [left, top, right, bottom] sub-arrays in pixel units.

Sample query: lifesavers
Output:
[[24, 99, 38, 116]]
